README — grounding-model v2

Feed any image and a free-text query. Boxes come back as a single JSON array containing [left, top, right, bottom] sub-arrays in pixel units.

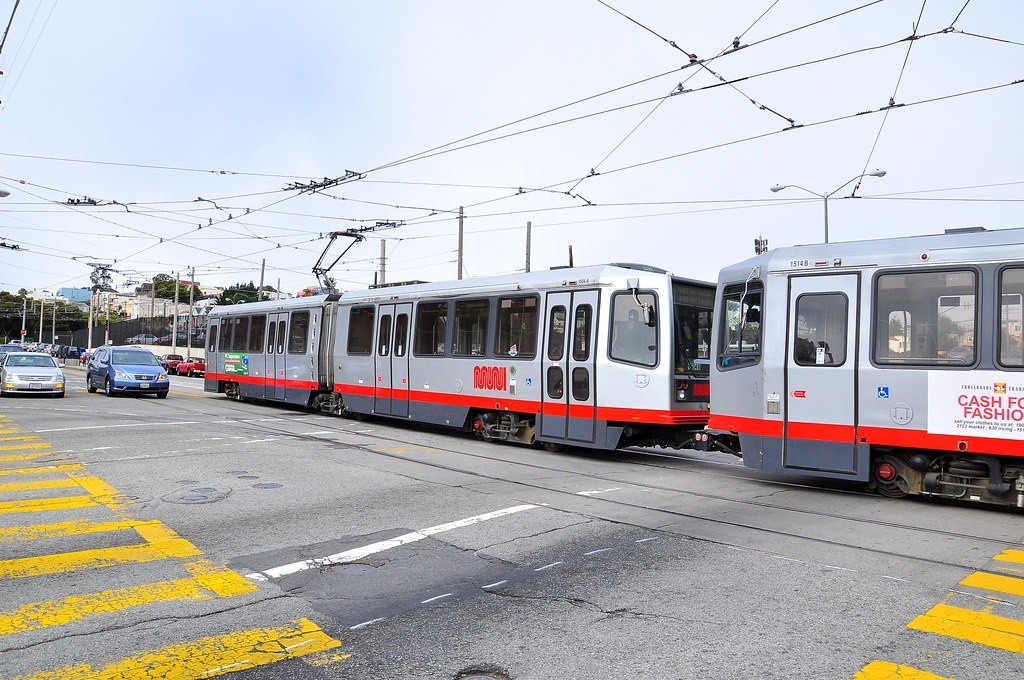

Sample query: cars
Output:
[[86, 345, 170, 399], [9, 339, 84, 359], [0, 344, 24, 364], [1, 352, 65, 398], [80, 351, 92, 363]]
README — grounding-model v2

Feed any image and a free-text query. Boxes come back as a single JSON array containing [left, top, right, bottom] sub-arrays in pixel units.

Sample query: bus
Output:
[[201, 222, 1024, 512]]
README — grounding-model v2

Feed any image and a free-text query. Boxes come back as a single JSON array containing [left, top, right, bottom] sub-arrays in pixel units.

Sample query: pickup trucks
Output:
[[176, 357, 206, 377], [161, 354, 183, 374]]
